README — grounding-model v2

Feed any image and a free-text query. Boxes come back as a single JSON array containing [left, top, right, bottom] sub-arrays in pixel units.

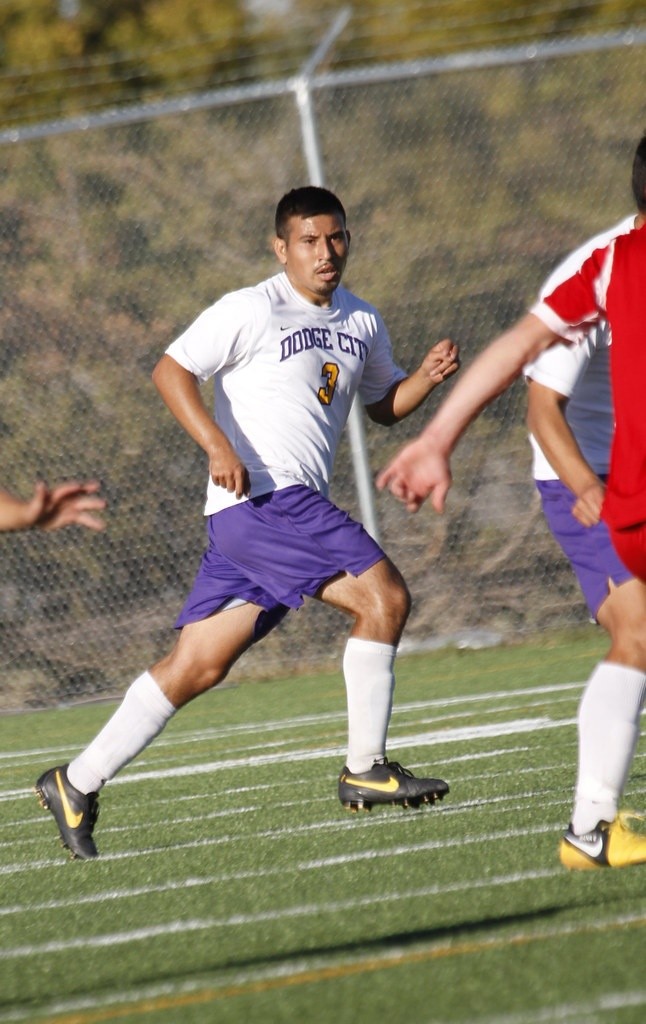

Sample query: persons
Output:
[[376, 223, 645, 585], [0, 481, 108, 532], [35, 185, 461, 863], [521, 134, 646, 870]]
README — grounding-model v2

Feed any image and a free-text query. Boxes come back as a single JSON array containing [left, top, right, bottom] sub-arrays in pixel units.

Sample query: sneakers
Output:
[[35, 760, 101, 863], [338, 757, 449, 813], [560, 809, 646, 870]]
